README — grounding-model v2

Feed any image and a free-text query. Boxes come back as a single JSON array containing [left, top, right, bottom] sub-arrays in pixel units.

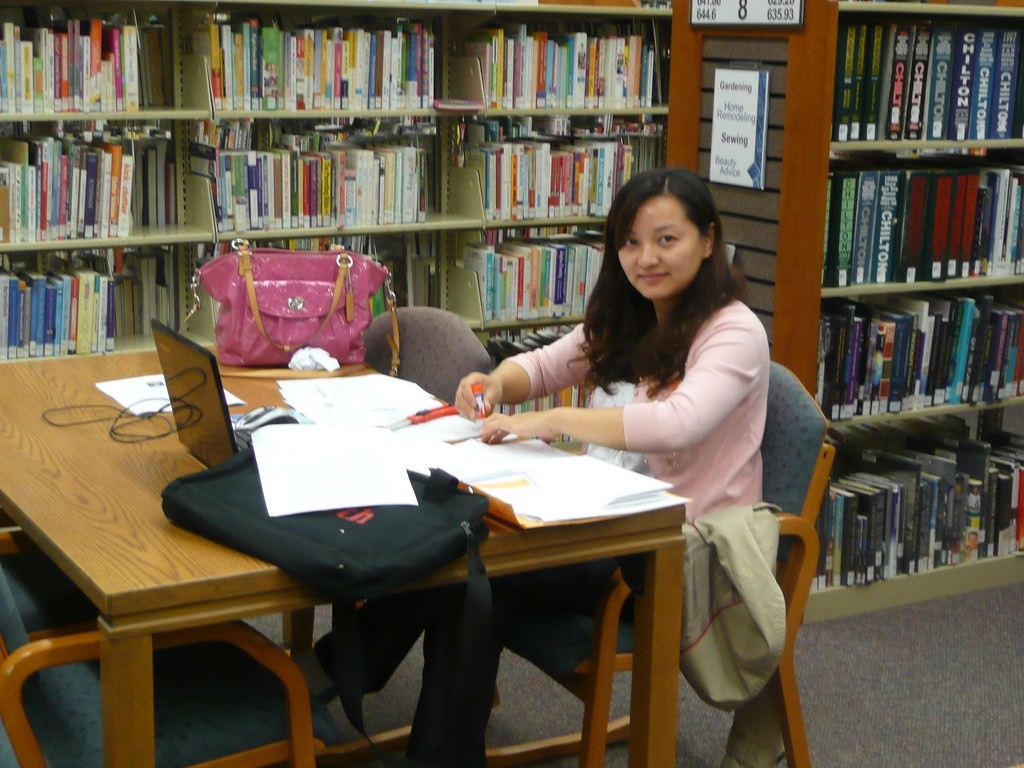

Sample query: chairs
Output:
[[505, 359, 837, 768], [364, 307, 491, 407], [0, 531, 324, 768]]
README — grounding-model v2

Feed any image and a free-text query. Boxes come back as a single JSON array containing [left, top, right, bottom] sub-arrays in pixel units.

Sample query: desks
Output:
[[0, 345, 686, 768]]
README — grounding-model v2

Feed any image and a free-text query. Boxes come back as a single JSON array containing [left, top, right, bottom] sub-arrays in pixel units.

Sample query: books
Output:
[[0, 0, 665, 444], [811, 22, 1024, 595]]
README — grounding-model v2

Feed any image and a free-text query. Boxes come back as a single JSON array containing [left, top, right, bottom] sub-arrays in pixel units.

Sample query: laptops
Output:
[[150, 317, 253, 468]]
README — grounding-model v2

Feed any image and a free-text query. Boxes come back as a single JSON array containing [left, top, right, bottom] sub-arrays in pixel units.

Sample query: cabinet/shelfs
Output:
[[0, 0, 1024, 625]]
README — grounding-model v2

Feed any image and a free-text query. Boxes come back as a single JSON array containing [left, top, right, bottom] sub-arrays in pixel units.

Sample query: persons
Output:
[[246, 169, 770, 766]]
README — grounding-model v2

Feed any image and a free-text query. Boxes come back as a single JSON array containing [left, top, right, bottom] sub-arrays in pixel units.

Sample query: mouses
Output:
[[235, 405, 301, 432]]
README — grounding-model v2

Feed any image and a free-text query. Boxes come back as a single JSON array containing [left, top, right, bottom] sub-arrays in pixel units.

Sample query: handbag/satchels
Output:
[[159, 441, 490, 605], [192, 240, 390, 367]]
